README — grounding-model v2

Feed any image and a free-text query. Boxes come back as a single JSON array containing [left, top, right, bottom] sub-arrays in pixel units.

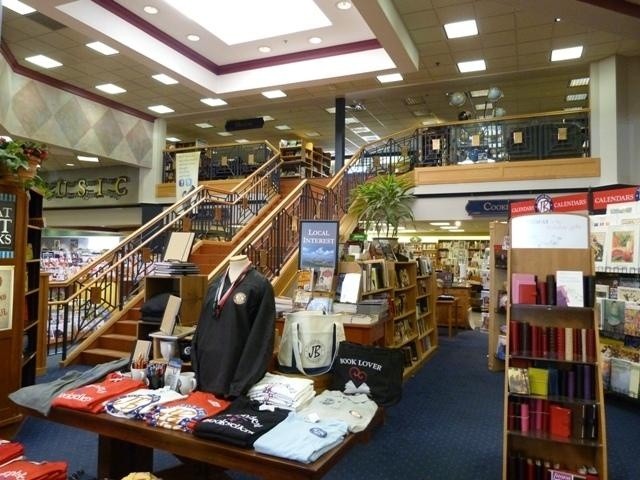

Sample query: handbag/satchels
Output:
[[329, 341, 407, 408], [276, 312, 346, 374]]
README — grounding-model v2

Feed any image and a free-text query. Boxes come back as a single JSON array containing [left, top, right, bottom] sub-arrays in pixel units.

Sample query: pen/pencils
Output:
[[132, 353, 148, 369], [148, 363, 167, 375]]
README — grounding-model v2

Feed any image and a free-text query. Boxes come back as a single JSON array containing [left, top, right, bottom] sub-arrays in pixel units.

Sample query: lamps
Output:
[[449, 84, 506, 120]]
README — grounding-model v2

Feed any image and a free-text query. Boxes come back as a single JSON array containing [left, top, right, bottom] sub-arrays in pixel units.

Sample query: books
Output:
[[590, 200, 640, 399], [492, 243, 603, 479], [154, 258, 201, 276], [272, 265, 337, 325], [337, 238, 436, 371], [128, 339, 153, 373], [162, 230, 195, 263], [159, 294, 183, 336], [436, 239, 489, 333]]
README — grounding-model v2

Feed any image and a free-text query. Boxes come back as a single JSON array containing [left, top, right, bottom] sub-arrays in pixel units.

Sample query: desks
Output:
[[272, 315, 394, 346], [436, 297, 460, 338], [7, 384, 383, 480]]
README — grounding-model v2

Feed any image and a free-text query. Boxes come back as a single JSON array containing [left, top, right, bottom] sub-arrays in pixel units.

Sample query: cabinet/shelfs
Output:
[[0, 184, 45, 427], [398, 240, 490, 310], [279, 142, 331, 178], [339, 259, 438, 382], [490, 212, 640, 480]]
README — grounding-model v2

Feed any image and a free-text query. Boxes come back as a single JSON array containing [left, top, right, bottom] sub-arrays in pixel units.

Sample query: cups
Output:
[[160, 339, 177, 361], [148, 374, 165, 390], [131, 364, 147, 381], [179, 372, 197, 395]]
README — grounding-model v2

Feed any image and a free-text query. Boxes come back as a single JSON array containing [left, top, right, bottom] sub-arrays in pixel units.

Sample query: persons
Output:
[[191, 252, 276, 403]]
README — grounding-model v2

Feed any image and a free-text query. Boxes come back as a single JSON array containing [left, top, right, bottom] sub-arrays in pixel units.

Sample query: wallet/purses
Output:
[[507, 360, 600, 440], [509, 451, 562, 479], [583, 274, 594, 308], [512, 273, 554, 305], [509, 320, 596, 364]]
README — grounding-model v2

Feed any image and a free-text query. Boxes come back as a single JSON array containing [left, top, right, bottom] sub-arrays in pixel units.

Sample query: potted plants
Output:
[[0, 139, 55, 201], [344, 170, 418, 255]]
